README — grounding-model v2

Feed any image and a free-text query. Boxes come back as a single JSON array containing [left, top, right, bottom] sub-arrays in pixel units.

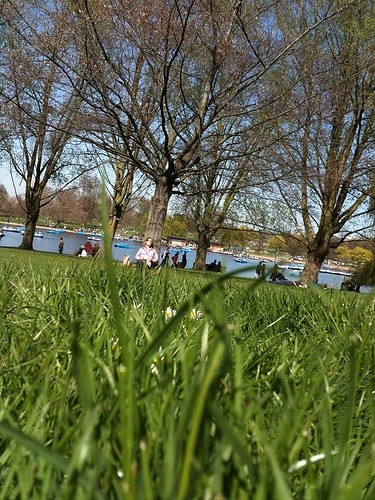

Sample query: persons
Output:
[[160, 246, 170, 267], [171, 251, 179, 268], [135, 237, 159, 269], [256, 262, 265, 280], [58, 237, 64, 254], [75, 241, 101, 257], [270, 264, 285, 281], [0, 226, 5, 241], [209, 259, 216, 272], [341, 277, 360, 292], [181, 252, 187, 268]]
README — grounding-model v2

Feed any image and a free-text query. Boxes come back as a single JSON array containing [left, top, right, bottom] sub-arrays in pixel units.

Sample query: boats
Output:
[[46, 230, 60, 235], [232, 257, 248, 263], [113, 243, 135, 249], [87, 236, 101, 241], [20, 230, 43, 239], [2, 226, 25, 233], [168, 244, 193, 253]]
